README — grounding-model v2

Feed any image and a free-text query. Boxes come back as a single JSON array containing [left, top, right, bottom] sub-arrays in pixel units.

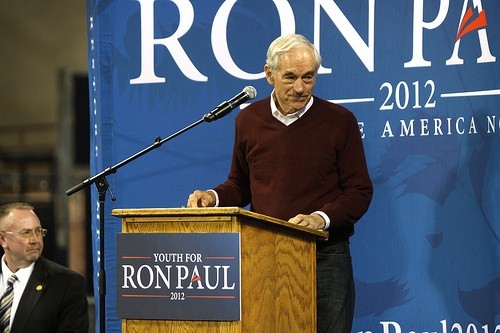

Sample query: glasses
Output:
[[1, 229, 47, 238]]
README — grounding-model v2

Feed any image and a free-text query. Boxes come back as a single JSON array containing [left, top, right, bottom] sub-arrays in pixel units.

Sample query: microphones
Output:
[[203, 86, 257, 123]]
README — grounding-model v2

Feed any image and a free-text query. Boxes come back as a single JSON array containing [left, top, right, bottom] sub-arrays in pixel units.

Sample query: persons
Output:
[[188, 33, 375, 333], [0, 202, 89, 333]]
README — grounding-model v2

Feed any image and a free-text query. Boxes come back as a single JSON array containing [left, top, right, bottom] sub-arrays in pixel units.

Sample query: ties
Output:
[[0, 275, 19, 333]]
